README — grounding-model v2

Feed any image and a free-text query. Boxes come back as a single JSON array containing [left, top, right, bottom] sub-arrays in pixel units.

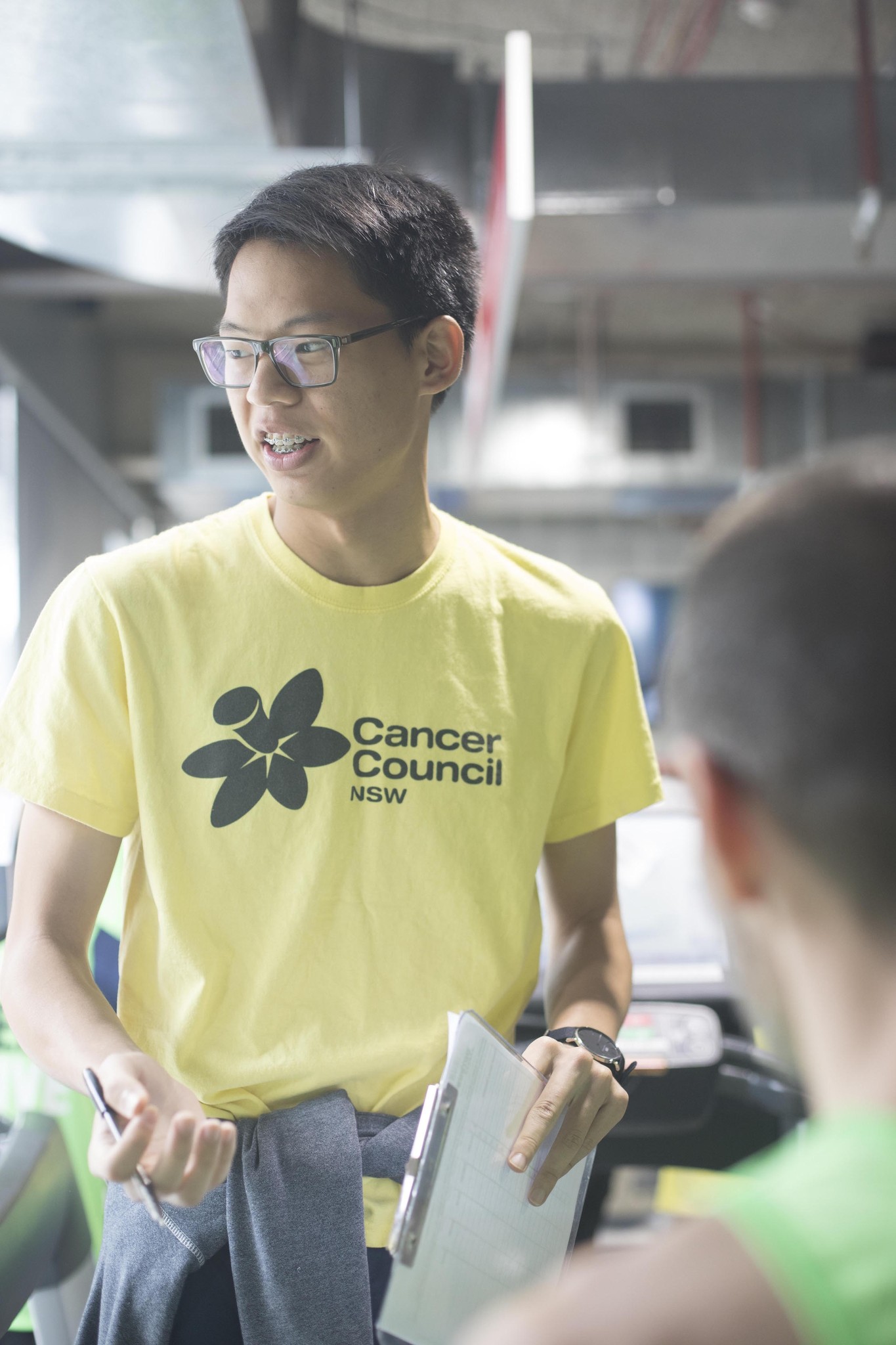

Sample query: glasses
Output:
[[190, 315, 418, 390]]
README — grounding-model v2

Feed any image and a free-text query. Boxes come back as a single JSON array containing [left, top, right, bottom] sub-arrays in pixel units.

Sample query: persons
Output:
[[0, 164, 667, 1345], [455, 435, 896, 1345]]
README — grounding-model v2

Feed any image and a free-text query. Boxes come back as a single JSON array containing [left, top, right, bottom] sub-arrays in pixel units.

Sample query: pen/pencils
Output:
[[82, 1067, 162, 1221]]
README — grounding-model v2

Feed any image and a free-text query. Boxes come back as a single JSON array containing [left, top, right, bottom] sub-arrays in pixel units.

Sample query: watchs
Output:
[[543, 1027, 637, 1086]]
